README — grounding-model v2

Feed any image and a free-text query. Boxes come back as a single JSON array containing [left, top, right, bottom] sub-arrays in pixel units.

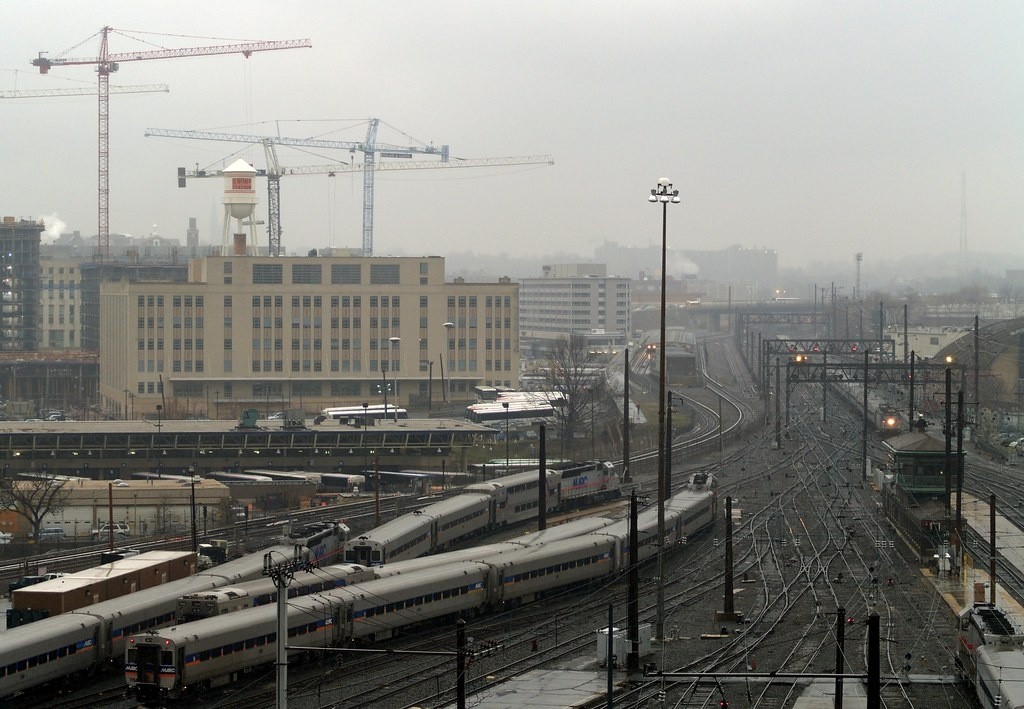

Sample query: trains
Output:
[[0, 454, 725, 709], [776, 334, 808, 365], [823, 364, 902, 434]]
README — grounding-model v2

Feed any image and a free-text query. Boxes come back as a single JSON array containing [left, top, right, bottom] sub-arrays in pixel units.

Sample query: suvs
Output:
[[89, 523, 131, 541]]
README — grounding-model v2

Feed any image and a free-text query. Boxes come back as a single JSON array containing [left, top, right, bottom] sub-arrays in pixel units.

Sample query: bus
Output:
[[320, 383, 566, 424]]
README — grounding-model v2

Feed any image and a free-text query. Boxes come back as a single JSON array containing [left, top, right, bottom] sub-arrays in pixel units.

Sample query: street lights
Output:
[[443, 322, 456, 405], [389, 337, 402, 423], [647, 176, 682, 640], [502, 402, 510, 476]]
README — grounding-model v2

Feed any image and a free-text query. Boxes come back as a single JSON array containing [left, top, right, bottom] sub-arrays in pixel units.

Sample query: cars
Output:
[[24, 407, 77, 422], [27, 528, 67, 542], [0, 531, 14, 544]]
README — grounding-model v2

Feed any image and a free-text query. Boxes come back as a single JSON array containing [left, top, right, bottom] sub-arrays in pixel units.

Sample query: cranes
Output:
[[0, 26, 557, 259]]
